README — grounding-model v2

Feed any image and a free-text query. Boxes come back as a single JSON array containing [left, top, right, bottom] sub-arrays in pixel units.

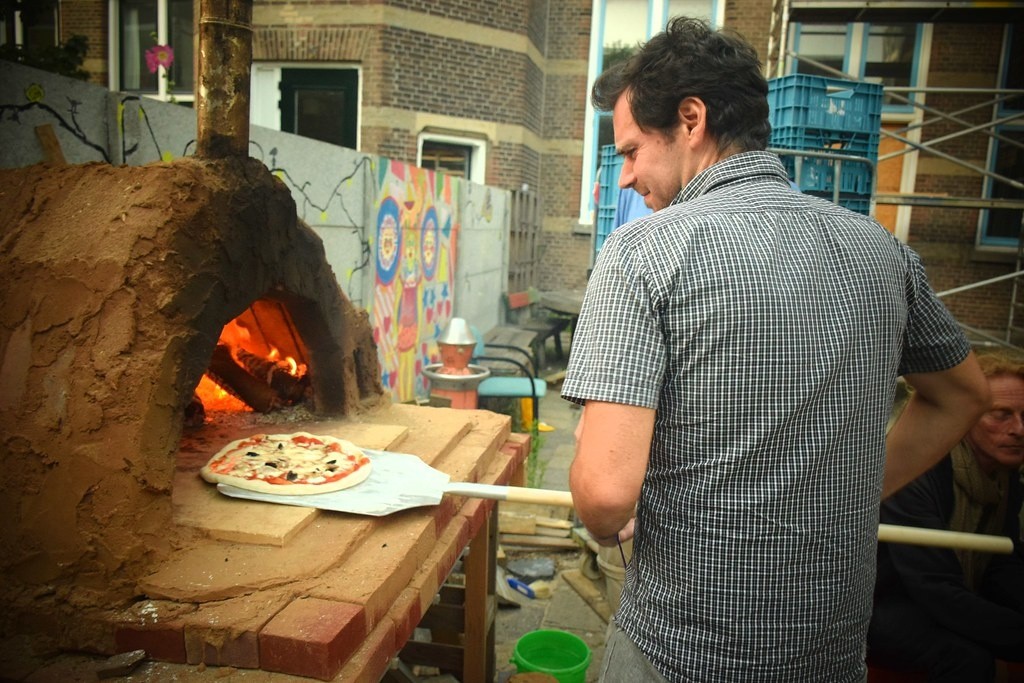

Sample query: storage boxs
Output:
[[594, 74, 884, 267]]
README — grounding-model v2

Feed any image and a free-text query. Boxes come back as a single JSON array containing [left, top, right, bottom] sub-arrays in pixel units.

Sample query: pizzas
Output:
[[199, 431, 373, 496]]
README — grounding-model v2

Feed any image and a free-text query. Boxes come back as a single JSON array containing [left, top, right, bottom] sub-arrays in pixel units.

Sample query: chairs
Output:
[[474, 341, 547, 452], [502, 286, 570, 369]]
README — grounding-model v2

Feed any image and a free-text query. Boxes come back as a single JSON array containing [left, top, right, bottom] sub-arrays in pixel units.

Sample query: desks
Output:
[[541, 290, 588, 343], [472, 323, 541, 377]]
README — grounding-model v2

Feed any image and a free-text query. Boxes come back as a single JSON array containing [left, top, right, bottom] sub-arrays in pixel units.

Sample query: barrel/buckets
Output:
[[510, 629, 592, 683], [595, 553, 626, 616]]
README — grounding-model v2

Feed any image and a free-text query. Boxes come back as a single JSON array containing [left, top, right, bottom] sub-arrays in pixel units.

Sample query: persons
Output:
[[866, 350, 1024, 683], [561, 17, 990, 683]]
